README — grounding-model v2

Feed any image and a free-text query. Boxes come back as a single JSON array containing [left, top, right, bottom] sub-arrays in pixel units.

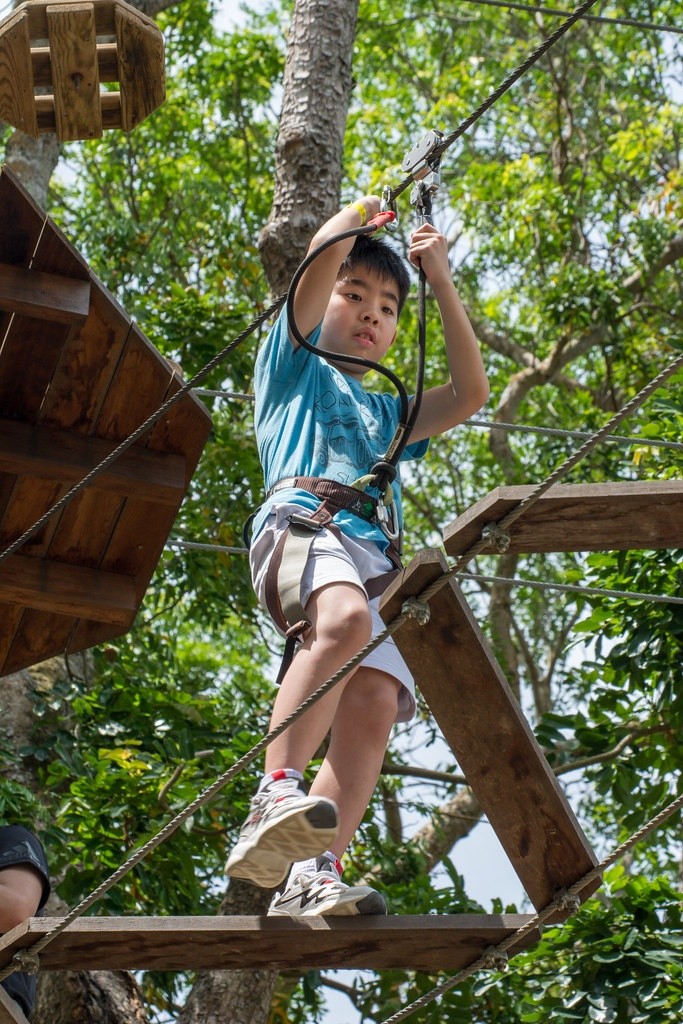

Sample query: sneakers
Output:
[[223, 788, 341, 889], [266, 874, 386, 917]]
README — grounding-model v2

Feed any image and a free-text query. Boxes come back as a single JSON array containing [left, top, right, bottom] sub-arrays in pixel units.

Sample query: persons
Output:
[[0, 824, 52, 1024], [221, 195, 489, 915]]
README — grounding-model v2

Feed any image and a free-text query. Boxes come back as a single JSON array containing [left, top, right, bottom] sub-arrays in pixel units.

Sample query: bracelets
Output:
[[343, 201, 367, 228]]
